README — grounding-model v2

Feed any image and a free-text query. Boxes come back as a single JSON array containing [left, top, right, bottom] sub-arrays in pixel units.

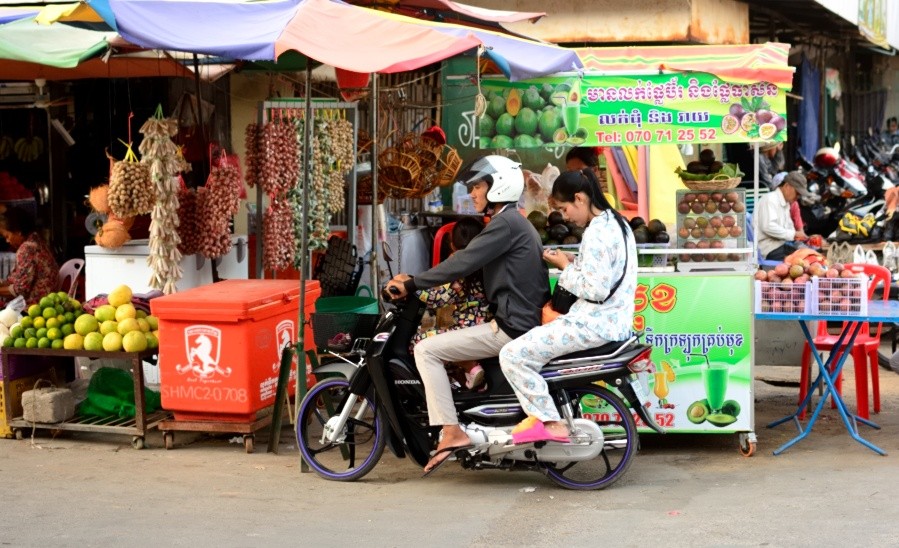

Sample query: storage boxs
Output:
[[753, 272, 870, 317], [148, 278, 322, 423]]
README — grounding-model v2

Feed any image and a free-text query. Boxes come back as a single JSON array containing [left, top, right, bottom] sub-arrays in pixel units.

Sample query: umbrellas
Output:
[[335, 0, 548, 430], [0, 7, 151, 68], [30, 0, 588, 473]]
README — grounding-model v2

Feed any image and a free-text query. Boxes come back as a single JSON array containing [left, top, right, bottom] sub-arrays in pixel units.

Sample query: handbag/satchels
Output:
[[551, 282, 578, 314]]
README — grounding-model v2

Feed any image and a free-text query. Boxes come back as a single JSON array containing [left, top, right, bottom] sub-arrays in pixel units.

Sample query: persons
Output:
[[751, 131, 809, 269], [0, 209, 60, 317], [548, 145, 617, 218], [882, 117, 899, 146], [384, 153, 551, 476], [393, 213, 494, 388], [498, 166, 638, 443]]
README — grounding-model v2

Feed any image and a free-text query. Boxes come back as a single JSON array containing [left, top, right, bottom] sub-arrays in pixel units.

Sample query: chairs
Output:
[[796, 263, 891, 425], [58, 258, 84, 300]]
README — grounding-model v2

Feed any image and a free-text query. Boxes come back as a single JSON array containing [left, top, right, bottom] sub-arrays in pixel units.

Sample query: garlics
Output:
[[176, 114, 354, 270], [106, 159, 154, 219]]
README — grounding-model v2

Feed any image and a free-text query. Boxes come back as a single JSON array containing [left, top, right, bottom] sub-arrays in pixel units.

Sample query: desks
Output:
[[1, 346, 175, 450], [754, 300, 899, 457]]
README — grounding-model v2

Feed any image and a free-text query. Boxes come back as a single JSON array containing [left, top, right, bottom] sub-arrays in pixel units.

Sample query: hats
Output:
[[785, 171, 811, 198], [773, 171, 788, 189]]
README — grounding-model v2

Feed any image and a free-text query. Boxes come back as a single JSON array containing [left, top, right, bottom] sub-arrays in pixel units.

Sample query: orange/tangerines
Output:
[[2, 291, 82, 349]]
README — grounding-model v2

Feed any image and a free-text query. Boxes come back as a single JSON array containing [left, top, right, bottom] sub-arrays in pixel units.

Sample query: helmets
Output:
[[454, 155, 526, 203]]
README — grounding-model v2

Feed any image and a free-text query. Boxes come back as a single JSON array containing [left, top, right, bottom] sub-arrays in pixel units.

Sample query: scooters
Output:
[[776, 123, 899, 242], [301, 240, 654, 490]]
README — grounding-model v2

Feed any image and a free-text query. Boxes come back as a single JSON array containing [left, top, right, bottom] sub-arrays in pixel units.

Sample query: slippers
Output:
[[422, 445, 477, 477], [512, 417, 570, 443]]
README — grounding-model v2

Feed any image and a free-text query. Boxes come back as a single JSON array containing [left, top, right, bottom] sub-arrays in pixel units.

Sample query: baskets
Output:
[[314, 237, 362, 296], [357, 129, 462, 206], [754, 279, 812, 316], [308, 312, 380, 351], [812, 276, 868, 317], [683, 174, 741, 190]]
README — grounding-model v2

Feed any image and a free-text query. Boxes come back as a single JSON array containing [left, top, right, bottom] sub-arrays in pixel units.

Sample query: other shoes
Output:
[[465, 365, 484, 388]]
